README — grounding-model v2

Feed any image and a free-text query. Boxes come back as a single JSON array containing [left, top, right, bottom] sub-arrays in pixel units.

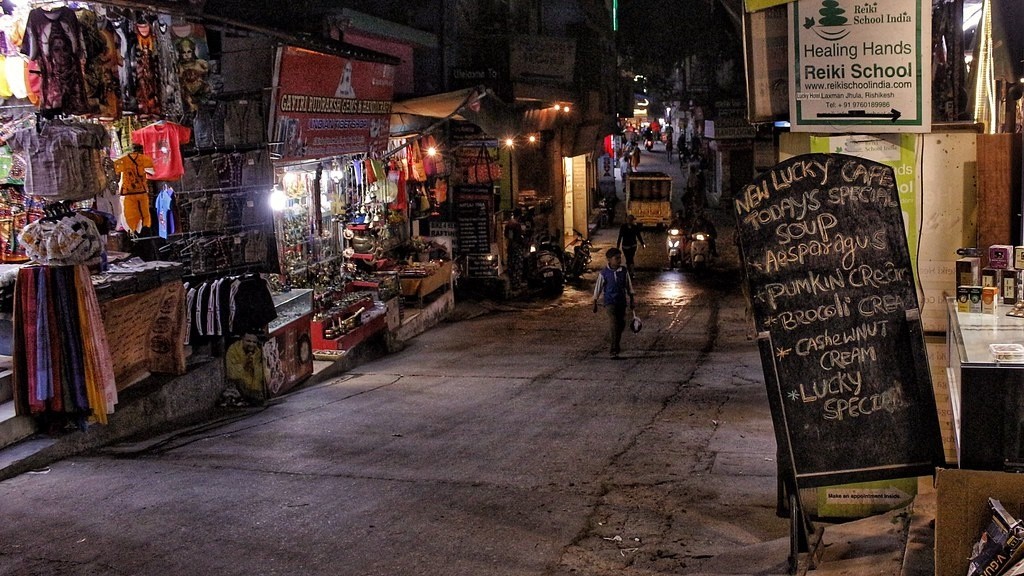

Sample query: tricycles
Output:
[[626, 172, 673, 230]]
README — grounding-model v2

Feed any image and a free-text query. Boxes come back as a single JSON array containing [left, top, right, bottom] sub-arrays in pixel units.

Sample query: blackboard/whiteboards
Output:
[[455, 183, 499, 278], [729, 150, 946, 490]]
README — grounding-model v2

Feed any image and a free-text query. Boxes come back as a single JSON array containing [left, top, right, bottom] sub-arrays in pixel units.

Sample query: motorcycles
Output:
[[643, 132, 654, 150]]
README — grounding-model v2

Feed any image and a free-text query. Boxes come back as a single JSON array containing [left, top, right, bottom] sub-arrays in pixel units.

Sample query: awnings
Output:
[[380, 84, 581, 159]]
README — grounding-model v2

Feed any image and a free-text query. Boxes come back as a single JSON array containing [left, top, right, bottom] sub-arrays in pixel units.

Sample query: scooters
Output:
[[660, 133, 667, 145], [571, 229, 593, 287], [689, 232, 711, 274], [530, 228, 565, 301], [666, 228, 688, 270]]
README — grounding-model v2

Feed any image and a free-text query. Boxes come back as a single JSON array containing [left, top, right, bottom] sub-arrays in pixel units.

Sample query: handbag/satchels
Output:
[[629, 308, 643, 334]]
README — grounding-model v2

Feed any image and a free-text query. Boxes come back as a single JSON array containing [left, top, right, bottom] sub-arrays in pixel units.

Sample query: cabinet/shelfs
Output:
[[945, 297, 1024, 472]]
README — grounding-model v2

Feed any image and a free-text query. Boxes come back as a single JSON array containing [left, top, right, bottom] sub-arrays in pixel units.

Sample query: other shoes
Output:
[[609, 352, 617, 360]]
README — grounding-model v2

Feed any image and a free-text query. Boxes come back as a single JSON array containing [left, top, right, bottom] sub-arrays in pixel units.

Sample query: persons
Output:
[[593, 248, 635, 360], [620, 117, 709, 214], [226, 331, 264, 401], [617, 215, 645, 279], [667, 210, 718, 258]]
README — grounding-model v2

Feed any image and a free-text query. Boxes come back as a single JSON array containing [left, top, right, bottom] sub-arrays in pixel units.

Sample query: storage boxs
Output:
[[956, 245, 1024, 314], [932, 465, 1024, 576], [816, 476, 916, 518]]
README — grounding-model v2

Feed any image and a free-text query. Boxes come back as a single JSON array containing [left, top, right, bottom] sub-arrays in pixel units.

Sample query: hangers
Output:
[[0, 0, 269, 290]]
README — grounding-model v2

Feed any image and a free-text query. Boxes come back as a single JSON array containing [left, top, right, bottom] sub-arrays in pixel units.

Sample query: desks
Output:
[[399, 259, 453, 308]]
